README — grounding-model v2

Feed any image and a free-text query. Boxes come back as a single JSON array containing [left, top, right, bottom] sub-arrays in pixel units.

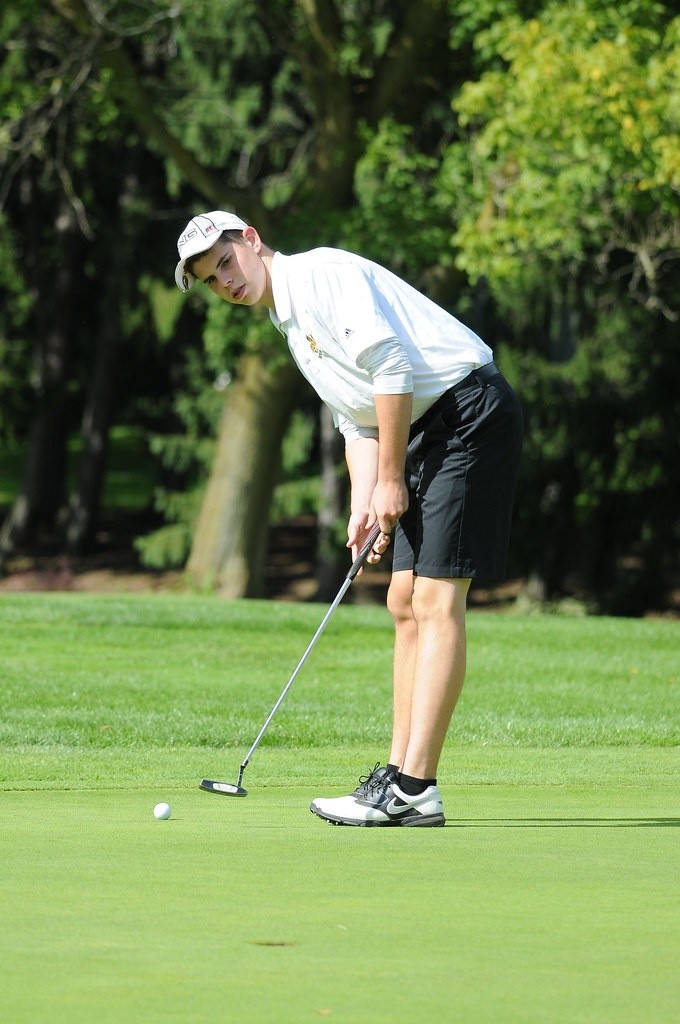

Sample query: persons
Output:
[[174, 210, 522, 826]]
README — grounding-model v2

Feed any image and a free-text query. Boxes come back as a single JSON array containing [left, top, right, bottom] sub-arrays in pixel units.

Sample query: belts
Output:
[[435, 362, 501, 409]]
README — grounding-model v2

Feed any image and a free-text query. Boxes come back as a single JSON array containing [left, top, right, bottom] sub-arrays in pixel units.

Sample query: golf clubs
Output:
[[198, 519, 381, 798]]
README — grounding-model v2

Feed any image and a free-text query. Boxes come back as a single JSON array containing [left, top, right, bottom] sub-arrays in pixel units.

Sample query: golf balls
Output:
[[153, 802, 171, 820]]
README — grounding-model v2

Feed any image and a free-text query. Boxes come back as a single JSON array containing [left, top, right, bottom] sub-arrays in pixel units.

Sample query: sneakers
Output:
[[310, 761, 447, 827]]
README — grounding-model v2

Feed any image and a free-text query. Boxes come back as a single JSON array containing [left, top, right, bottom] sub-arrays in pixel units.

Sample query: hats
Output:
[[175, 211, 249, 293]]
